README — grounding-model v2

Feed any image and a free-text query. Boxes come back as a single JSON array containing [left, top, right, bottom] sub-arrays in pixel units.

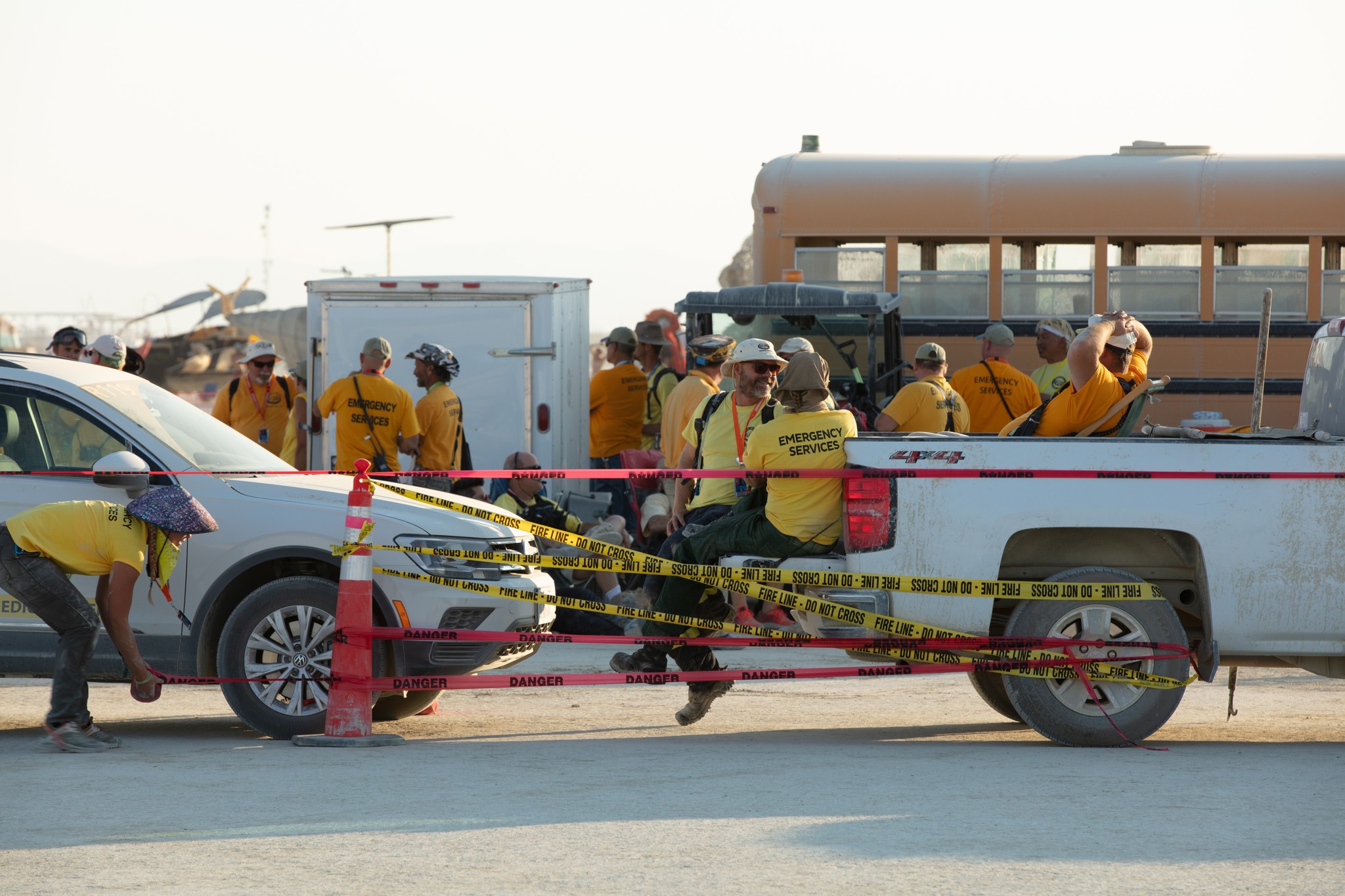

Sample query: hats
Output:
[[601, 327, 814, 378], [288, 358, 307, 379], [363, 337, 392, 359], [86, 334, 126, 360], [128, 483, 219, 534], [405, 343, 460, 376], [236, 341, 283, 364], [916, 314, 1137, 362]]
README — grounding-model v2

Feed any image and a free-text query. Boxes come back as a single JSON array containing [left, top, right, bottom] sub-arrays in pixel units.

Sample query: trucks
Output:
[[302, 275, 593, 499]]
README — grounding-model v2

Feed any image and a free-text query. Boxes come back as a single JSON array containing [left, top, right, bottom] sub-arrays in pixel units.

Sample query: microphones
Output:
[[531, 479, 546, 484]]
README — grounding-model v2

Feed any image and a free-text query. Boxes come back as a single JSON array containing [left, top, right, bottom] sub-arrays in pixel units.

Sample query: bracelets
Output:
[[135, 670, 152, 685]]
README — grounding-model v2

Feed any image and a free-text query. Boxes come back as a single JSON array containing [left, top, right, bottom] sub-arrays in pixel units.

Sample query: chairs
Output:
[[490, 450, 665, 591], [896, 295, 1117, 312], [0, 404, 23, 472], [1076, 375, 1170, 438]]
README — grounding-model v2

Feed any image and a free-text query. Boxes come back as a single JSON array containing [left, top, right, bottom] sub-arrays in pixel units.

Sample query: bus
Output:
[[749, 139, 1345, 429]]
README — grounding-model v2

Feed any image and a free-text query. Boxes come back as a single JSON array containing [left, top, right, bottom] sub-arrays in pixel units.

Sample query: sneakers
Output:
[[576, 517, 623, 558], [82, 722, 123, 749], [758, 607, 795, 626], [734, 606, 760, 628], [37, 721, 106, 753], [610, 650, 667, 673], [676, 676, 735, 727]]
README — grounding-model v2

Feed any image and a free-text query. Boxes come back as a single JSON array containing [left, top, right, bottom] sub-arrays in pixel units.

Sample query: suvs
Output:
[[1, 348, 557, 742]]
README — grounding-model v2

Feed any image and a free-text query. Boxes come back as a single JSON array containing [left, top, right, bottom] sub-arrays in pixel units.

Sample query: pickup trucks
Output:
[[717, 317, 1345, 747]]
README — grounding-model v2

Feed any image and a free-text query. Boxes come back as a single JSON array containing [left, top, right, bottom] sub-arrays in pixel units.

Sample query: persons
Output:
[[312, 337, 420, 483], [1028, 318, 1080, 400], [589, 309, 868, 650], [450, 451, 635, 607], [609, 352, 858, 727], [948, 320, 1042, 434], [908, 309, 1153, 440], [280, 359, 307, 476], [0, 325, 130, 471], [405, 343, 464, 492], [874, 343, 970, 434], [208, 341, 297, 456], [0, 484, 220, 752]]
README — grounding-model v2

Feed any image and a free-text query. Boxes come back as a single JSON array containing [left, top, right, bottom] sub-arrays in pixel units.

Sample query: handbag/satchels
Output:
[[461, 429, 473, 470], [620, 450, 664, 490], [412, 465, 451, 493]]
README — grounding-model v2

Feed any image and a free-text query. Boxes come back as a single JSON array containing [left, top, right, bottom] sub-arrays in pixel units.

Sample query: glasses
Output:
[[250, 360, 275, 368], [606, 341, 615, 346], [519, 465, 541, 470], [741, 361, 780, 376], [783, 355, 793, 361], [473, 494, 491, 501], [88, 350, 96, 356], [55, 329, 87, 347]]
